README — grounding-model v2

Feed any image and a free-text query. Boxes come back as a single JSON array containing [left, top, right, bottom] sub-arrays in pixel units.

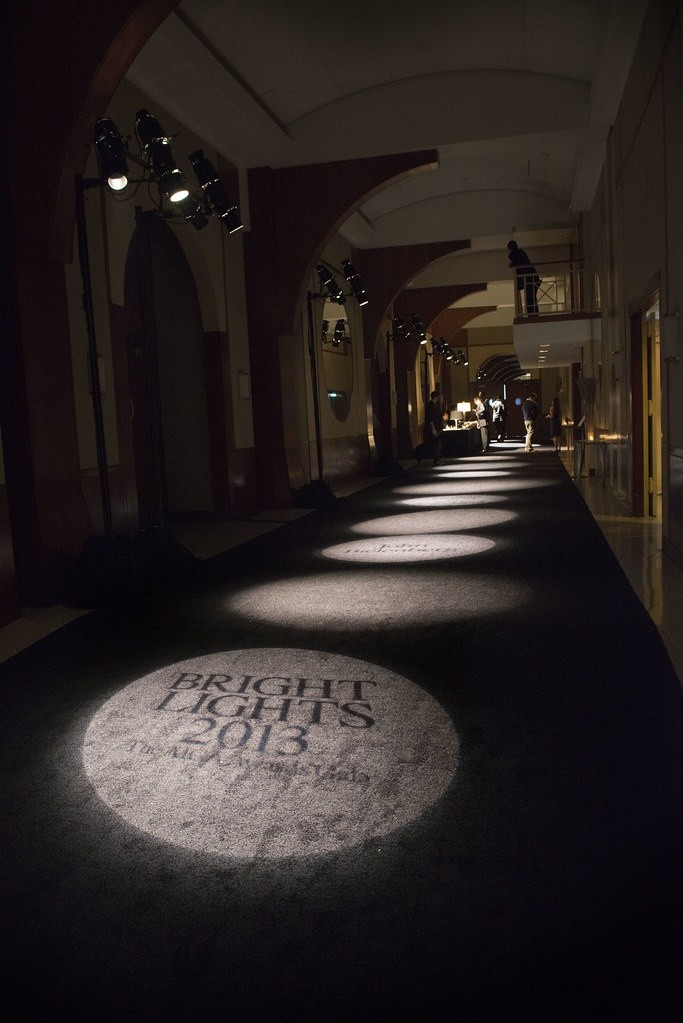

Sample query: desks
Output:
[[442, 421, 481, 455]]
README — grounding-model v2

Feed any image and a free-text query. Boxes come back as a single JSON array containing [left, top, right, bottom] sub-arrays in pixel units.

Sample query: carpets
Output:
[[0, 439, 683, 1023]]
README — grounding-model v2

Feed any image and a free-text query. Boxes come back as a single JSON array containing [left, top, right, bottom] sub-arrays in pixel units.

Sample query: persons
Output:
[[442, 410, 449, 428], [507, 240, 540, 317], [478, 391, 489, 411], [546, 398, 562, 451], [473, 396, 490, 453], [425, 391, 445, 465], [492, 394, 507, 442], [521, 392, 538, 450]]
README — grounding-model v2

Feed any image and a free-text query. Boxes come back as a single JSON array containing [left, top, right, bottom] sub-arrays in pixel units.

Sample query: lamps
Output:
[[340, 257, 369, 307], [478, 367, 487, 377], [136, 108, 245, 236], [476, 373, 482, 380], [450, 411, 464, 430], [332, 318, 345, 347], [456, 401, 471, 421], [315, 264, 346, 304], [321, 320, 329, 342], [94, 116, 131, 192], [394, 313, 469, 366]]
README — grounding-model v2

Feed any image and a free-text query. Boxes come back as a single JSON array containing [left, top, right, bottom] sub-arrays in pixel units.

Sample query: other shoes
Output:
[[553, 449, 562, 452], [479, 450, 488, 454], [525, 447, 534, 451], [432, 460, 444, 465]]
